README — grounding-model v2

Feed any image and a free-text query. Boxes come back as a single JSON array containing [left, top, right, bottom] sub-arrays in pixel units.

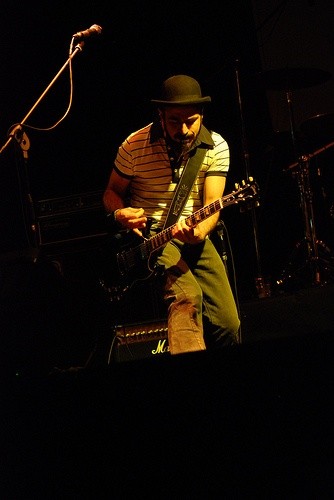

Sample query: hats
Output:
[[152, 75, 211, 105]]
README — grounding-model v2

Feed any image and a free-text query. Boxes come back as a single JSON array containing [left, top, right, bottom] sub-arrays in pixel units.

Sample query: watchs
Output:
[[110, 208, 121, 222]]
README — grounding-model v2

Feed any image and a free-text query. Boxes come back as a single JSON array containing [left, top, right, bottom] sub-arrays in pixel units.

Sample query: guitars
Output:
[[92, 174, 261, 309]]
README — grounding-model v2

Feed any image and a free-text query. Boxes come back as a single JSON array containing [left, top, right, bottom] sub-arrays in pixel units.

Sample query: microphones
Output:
[[72, 24, 102, 40]]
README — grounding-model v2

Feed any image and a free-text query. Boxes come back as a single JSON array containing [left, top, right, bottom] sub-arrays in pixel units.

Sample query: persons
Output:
[[103, 76, 241, 355]]
